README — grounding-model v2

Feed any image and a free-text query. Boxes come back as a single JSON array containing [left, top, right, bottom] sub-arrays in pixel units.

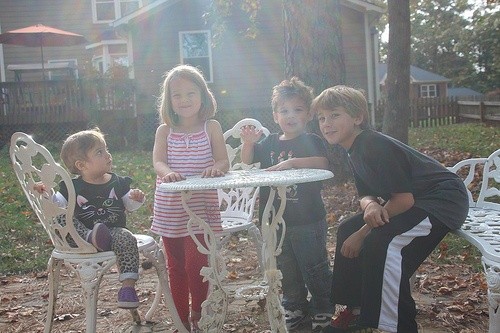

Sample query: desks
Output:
[[159, 168, 335, 333]]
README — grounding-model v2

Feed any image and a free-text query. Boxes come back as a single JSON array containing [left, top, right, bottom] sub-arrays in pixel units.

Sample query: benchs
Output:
[[440, 145, 500, 333]]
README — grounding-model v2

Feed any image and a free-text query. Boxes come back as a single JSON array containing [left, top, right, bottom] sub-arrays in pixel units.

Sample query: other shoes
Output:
[[116, 286, 141, 307], [171, 319, 203, 332], [88, 223, 114, 252]]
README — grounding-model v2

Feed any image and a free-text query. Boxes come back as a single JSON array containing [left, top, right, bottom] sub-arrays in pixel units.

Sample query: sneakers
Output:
[[321, 308, 362, 332], [283, 308, 313, 330], [312, 313, 332, 332]]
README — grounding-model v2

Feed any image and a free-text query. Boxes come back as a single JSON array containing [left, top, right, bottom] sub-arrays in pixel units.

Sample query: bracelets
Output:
[[365, 201, 376, 210]]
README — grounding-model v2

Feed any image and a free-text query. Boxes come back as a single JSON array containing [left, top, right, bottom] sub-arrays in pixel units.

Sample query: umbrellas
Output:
[[0, 23, 88, 114]]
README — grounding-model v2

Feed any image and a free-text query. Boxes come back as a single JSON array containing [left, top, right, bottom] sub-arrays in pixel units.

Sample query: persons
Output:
[[240, 78, 334, 329], [312, 85, 469, 333], [151, 66, 230, 333], [35, 130, 145, 308]]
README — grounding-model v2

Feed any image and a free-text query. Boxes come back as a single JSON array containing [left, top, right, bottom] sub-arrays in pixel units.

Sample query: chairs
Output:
[[10, 132, 166, 333], [203, 118, 271, 291]]
